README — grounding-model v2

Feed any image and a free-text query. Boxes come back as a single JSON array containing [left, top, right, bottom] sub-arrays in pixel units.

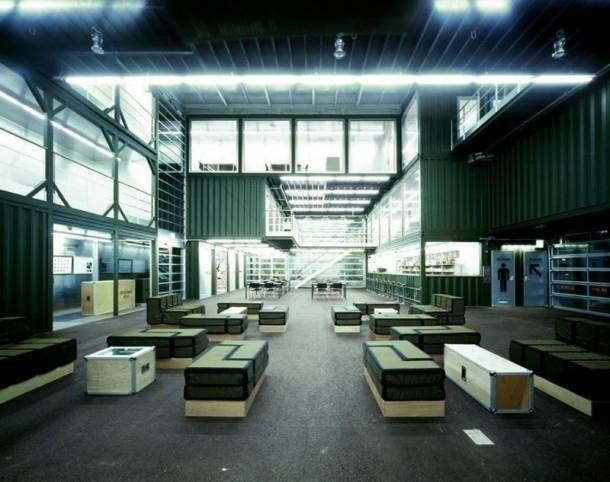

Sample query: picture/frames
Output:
[[52, 255, 74, 276]]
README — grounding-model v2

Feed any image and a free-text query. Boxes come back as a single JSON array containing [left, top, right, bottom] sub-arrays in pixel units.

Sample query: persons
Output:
[[497, 263, 510, 292]]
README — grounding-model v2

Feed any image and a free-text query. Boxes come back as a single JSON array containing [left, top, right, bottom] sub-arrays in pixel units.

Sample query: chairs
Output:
[[242, 277, 348, 300]]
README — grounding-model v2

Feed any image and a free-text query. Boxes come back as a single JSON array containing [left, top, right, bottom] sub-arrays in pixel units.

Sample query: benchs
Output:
[[551, 311, 608, 352], [2, 315, 80, 404], [143, 293, 207, 330], [178, 312, 251, 343], [181, 338, 272, 420], [105, 324, 211, 371], [408, 294, 468, 328], [213, 299, 260, 316], [506, 336, 608, 419], [388, 322, 484, 356], [368, 314, 435, 339], [358, 337, 448, 422], [257, 303, 292, 334], [329, 303, 364, 335], [352, 298, 404, 314]]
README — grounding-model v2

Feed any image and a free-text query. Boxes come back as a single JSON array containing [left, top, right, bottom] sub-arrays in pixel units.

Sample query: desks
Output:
[[82, 344, 158, 398], [372, 305, 399, 316], [441, 341, 536, 417], [220, 304, 248, 319]]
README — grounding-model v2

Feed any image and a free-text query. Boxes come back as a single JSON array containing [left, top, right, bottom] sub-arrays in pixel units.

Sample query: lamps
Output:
[[331, 29, 359, 62], [87, 23, 108, 60], [550, 28, 569, 61]]
